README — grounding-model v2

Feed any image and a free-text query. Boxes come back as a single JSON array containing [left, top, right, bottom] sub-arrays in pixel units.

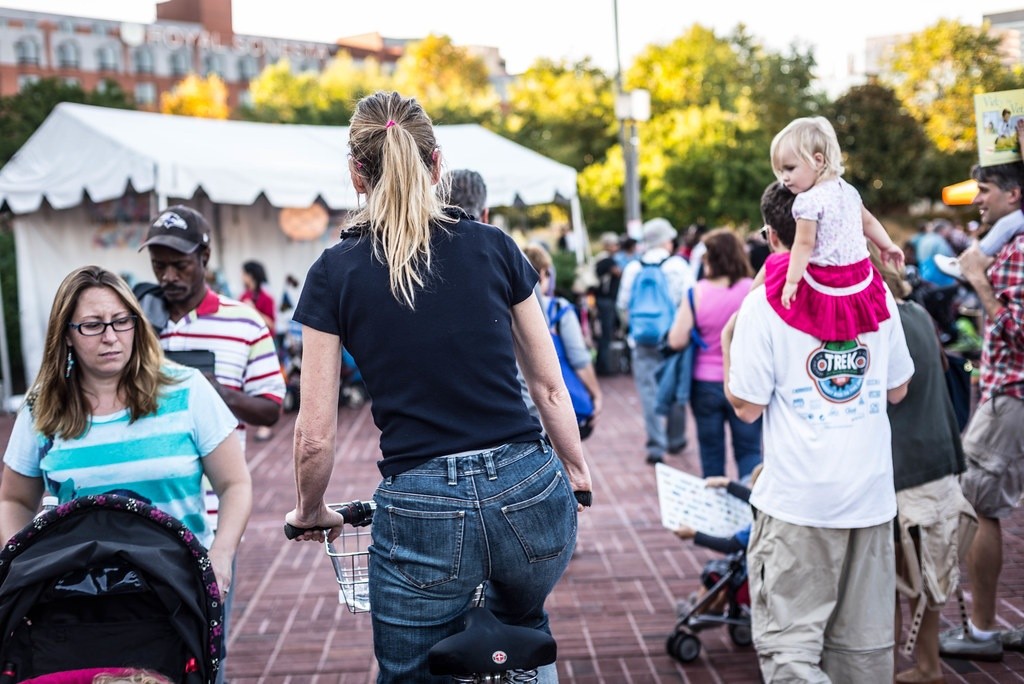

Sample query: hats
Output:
[[640, 217, 678, 247], [138, 205, 210, 252]]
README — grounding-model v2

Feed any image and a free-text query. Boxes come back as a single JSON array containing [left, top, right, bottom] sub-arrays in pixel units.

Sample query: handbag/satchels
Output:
[[548, 298, 593, 419]]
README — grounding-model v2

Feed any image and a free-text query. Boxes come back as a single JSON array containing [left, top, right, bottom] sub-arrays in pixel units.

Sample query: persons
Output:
[[438, 166, 602, 446], [749, 118, 907, 342], [138, 204, 289, 684], [584, 118, 1024, 684], [989, 108, 1017, 150], [0, 265, 255, 605], [201, 261, 368, 440], [720, 179, 917, 684], [283, 90, 594, 684]]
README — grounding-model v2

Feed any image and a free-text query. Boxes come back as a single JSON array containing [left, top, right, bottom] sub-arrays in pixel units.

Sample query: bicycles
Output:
[[283, 489, 592, 684]]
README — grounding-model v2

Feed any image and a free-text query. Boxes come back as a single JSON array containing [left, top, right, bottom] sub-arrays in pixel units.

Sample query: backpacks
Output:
[[629, 258, 675, 346]]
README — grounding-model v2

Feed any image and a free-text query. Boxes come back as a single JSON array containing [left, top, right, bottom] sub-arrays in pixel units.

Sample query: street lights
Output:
[[614, 86, 651, 231]]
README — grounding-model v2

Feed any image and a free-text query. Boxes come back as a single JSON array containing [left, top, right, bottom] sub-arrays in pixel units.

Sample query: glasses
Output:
[[759, 223, 776, 241], [70, 315, 138, 336]]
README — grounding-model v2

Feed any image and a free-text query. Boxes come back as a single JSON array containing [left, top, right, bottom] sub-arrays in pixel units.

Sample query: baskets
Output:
[[321, 502, 373, 614]]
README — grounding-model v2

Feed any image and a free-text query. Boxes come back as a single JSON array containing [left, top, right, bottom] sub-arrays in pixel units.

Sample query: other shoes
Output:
[[667, 441, 688, 454], [675, 591, 700, 619], [936, 627, 1004, 662], [1002, 629, 1024, 652], [647, 455, 663, 465], [934, 254, 968, 281]]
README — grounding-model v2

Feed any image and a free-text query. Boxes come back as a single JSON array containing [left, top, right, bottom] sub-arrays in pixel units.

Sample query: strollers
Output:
[[664, 547, 752, 664], [0, 489, 226, 684]]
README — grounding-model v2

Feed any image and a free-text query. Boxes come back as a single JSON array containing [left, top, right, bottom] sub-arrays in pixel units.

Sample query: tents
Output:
[[0, 100, 585, 400]]
